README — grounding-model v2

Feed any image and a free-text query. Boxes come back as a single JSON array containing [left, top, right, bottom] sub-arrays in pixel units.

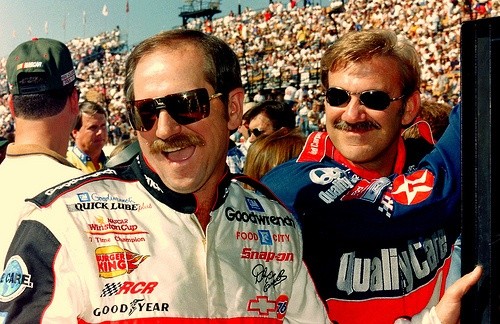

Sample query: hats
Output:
[[243, 102, 259, 116], [6, 38, 85, 95]]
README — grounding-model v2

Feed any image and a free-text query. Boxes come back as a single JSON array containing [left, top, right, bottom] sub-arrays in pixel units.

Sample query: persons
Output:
[[259, 30, 462, 324], [1, 0, 499, 182], [1, 30, 482, 324]]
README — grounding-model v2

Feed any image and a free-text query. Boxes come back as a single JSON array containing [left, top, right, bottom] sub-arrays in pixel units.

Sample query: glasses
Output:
[[325, 87, 405, 111], [248, 128, 276, 137], [126, 88, 223, 132], [242, 120, 250, 128]]
